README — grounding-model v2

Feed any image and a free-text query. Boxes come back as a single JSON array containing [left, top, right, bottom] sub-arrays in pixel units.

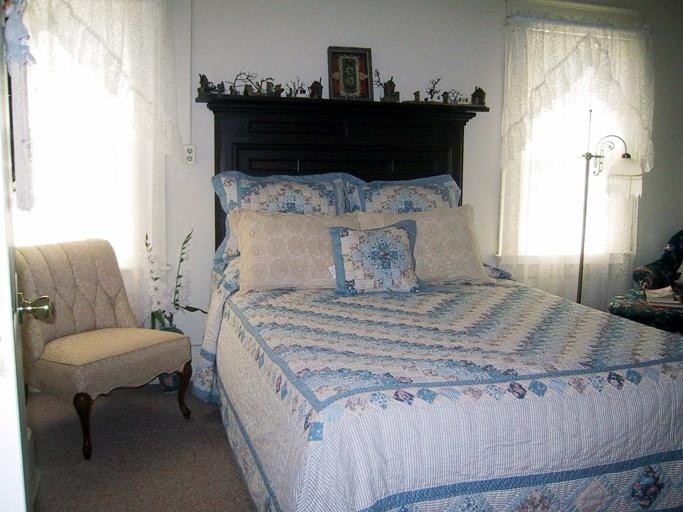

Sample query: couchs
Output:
[[608, 230, 683, 333], [14, 238, 192, 460]]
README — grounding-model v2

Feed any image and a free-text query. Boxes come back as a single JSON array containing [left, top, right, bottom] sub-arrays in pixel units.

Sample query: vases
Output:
[[159, 325, 191, 393]]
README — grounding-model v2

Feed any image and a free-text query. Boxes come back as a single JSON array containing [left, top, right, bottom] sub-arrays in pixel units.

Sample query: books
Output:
[[646, 285, 683, 308]]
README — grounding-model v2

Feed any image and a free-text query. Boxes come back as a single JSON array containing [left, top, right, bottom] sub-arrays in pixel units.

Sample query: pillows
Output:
[[339, 226, 421, 296], [329, 220, 416, 297], [211, 170, 367, 268], [356, 206, 497, 285], [340, 173, 461, 215], [228, 208, 361, 296]]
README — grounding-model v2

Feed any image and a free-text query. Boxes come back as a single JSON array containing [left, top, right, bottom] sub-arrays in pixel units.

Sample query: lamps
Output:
[[576, 135, 642, 304]]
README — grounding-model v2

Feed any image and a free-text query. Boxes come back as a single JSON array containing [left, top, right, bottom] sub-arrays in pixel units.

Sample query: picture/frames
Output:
[[327, 46, 374, 101]]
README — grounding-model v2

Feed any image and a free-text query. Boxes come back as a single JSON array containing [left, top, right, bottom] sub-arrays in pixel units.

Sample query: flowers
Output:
[[144, 228, 209, 326]]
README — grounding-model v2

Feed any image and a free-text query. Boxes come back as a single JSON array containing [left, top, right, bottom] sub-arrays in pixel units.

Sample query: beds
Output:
[[189, 266, 683, 512]]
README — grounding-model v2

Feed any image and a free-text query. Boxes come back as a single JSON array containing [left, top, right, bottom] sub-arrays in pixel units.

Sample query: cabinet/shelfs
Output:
[[195, 94, 490, 253]]
